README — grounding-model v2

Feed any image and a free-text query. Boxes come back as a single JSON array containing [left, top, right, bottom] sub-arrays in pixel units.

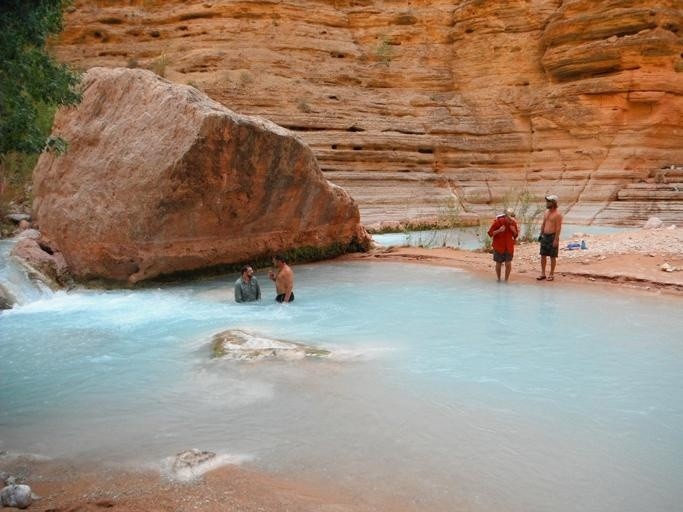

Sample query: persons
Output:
[[487, 209, 518, 282], [267, 253, 294, 303], [535, 195, 562, 281], [234, 264, 261, 303]]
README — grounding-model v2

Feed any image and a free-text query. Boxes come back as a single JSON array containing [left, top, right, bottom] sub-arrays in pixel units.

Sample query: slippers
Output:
[[547, 276, 554, 281], [537, 275, 546, 279]]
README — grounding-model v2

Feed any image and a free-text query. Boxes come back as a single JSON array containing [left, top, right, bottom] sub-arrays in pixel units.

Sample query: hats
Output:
[[505, 208, 515, 220], [545, 195, 557, 203]]
